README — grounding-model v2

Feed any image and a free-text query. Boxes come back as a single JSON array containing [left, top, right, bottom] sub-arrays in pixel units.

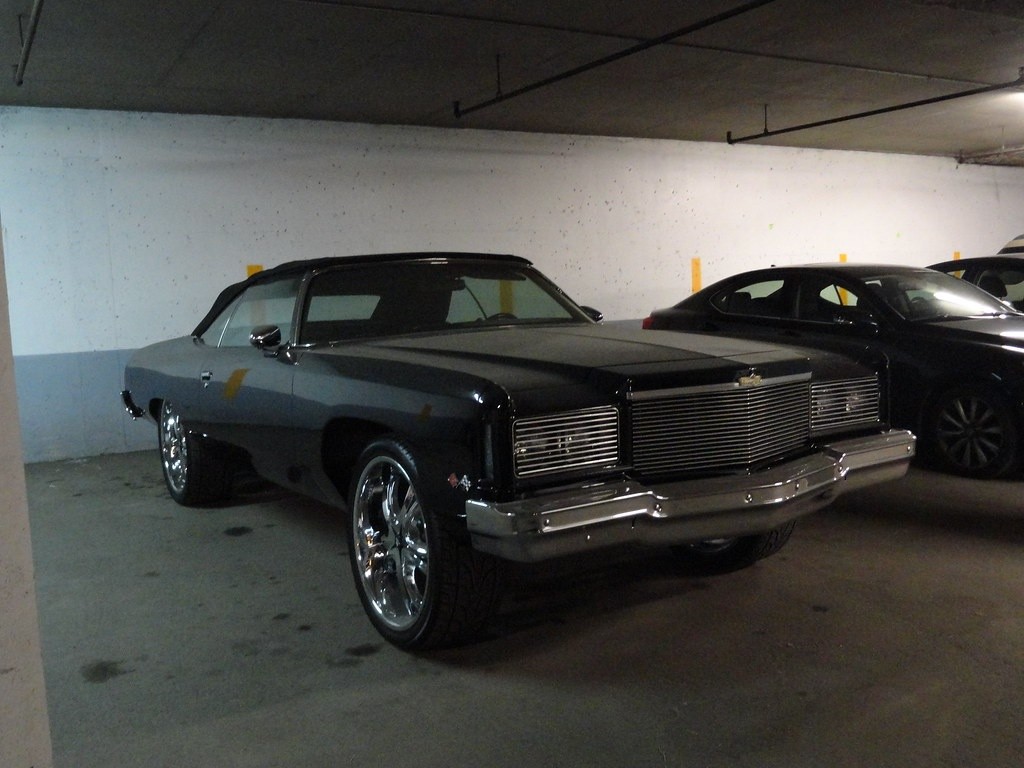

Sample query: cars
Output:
[[121, 250, 917, 656], [642, 261, 1024, 482], [902, 233, 1024, 315]]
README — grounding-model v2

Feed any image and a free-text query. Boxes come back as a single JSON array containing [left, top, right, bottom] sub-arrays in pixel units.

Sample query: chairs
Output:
[[398, 285, 453, 334], [724, 292, 752, 314], [979, 276, 1008, 300], [856, 284, 886, 313]]
